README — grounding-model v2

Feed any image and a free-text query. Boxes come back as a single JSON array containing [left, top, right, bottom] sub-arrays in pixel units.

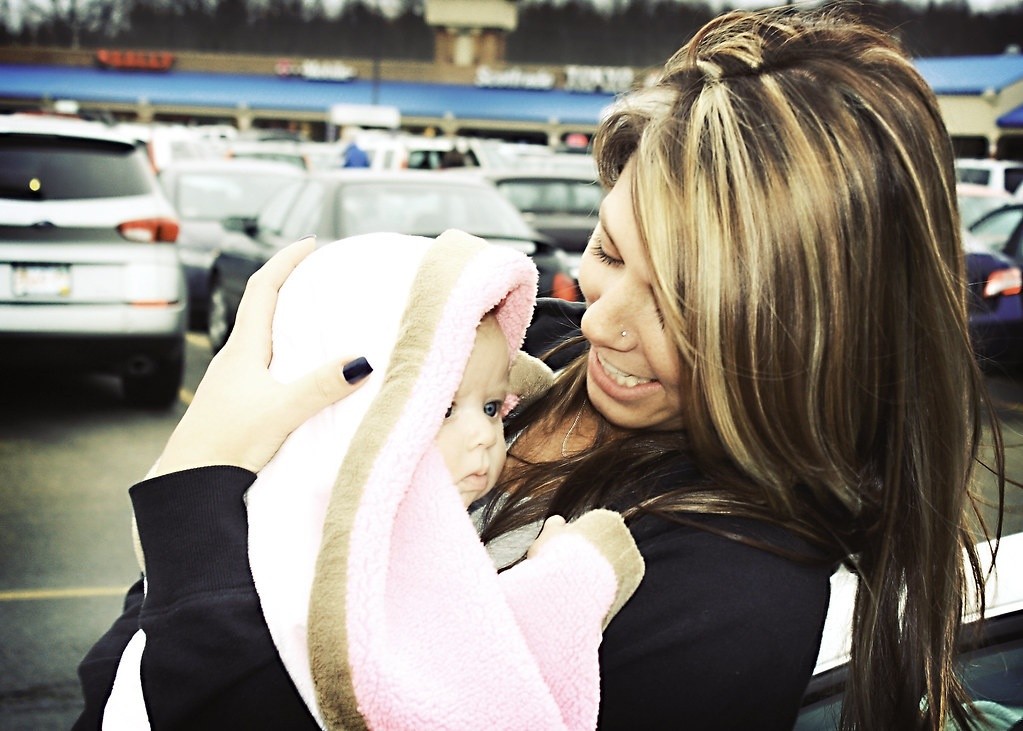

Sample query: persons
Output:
[[97, 217, 649, 731], [63, 0, 1023, 731], [336, 127, 464, 170]]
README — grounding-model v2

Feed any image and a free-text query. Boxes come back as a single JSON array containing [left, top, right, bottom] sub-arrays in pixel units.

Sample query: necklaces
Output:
[[561, 395, 594, 458]]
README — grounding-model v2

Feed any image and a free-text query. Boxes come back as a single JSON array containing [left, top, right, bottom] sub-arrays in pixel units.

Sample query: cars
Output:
[[0, 110, 1023, 421], [793, 531, 1022, 731]]
